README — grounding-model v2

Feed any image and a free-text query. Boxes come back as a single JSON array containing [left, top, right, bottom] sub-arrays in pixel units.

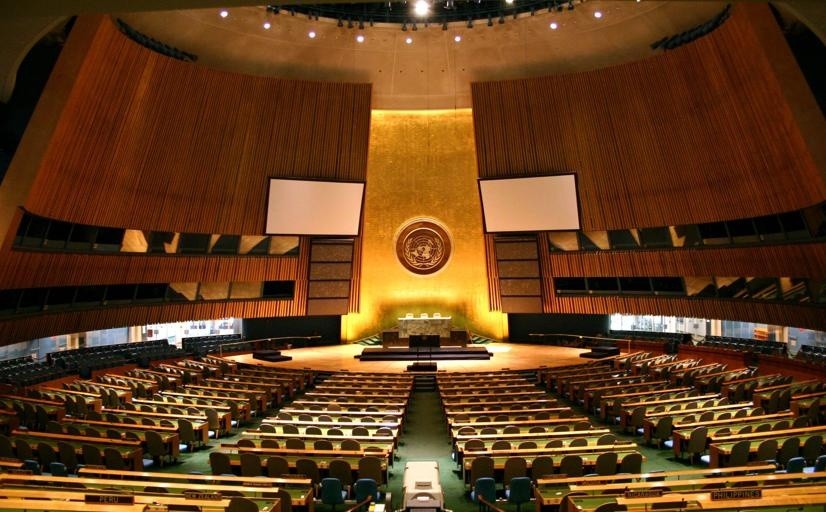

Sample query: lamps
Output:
[[413, 0, 431, 17]]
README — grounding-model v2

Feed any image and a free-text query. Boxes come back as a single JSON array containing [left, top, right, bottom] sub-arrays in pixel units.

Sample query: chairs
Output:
[[589, 350, 694, 367], [182, 355, 237, 371], [420, 312, 428, 318], [606, 327, 693, 350], [468, 476, 498, 512], [505, 476, 532, 512], [432, 313, 441, 318], [695, 334, 788, 368], [46, 338, 182, 372], [349, 478, 380, 512], [0, 355, 45, 372], [316, 370, 416, 476], [318, 477, 347, 512], [406, 313, 413, 318], [647, 368, 825, 473], [182, 334, 245, 352], [435, 369, 646, 474], [792, 343, 826, 366]]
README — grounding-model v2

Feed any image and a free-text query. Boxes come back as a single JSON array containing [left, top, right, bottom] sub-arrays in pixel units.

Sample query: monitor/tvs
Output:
[[261, 176, 365, 238], [478, 172, 583, 235]]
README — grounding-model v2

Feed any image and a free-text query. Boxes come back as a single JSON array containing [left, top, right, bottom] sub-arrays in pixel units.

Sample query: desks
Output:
[[532, 476, 825, 511]]
